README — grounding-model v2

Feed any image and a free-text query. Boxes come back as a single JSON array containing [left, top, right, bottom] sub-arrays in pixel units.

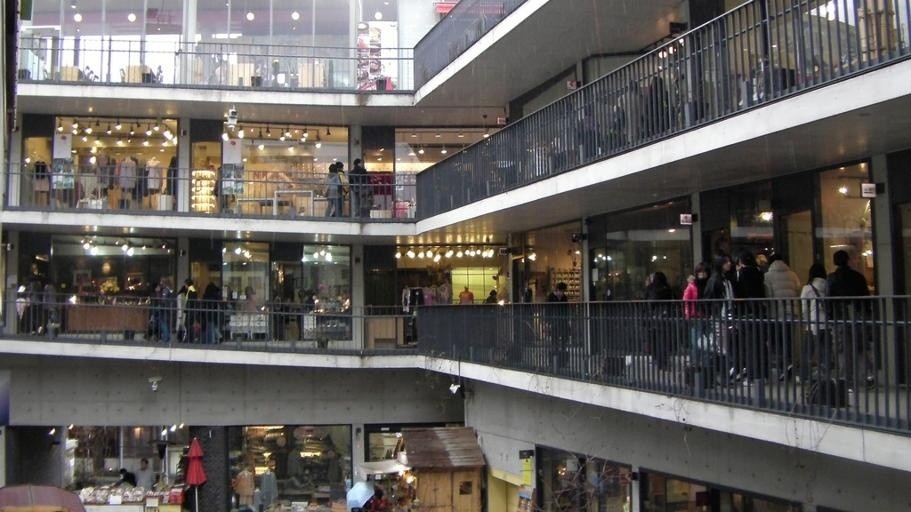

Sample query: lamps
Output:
[[160, 425, 168, 436], [395, 243, 495, 263], [220, 122, 322, 151], [394, 130, 489, 156], [47, 426, 55, 435], [57, 117, 177, 147]]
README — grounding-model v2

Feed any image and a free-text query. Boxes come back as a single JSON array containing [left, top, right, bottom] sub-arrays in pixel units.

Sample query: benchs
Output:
[[236, 189, 327, 216]]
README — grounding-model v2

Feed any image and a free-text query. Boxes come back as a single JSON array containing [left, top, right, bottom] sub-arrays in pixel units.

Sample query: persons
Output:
[[458, 286, 476, 305], [259, 471, 278, 506], [544, 283, 571, 367], [133, 458, 155, 493], [684, 252, 874, 388], [331, 161, 350, 216], [328, 452, 344, 482], [286, 444, 306, 481], [107, 468, 136, 492], [325, 164, 341, 217], [232, 467, 256, 506], [640, 272, 680, 371], [32, 152, 162, 190], [485, 290, 498, 347], [348, 158, 372, 218], [462, 73, 675, 201], [18, 275, 258, 344]]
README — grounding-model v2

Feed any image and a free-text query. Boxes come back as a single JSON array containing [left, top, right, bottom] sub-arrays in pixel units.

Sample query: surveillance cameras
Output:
[[148, 376, 164, 392], [227, 105, 238, 131]]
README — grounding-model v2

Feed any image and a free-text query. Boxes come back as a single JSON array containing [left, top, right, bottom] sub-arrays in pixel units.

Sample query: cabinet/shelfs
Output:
[[546, 264, 581, 298], [229, 314, 271, 341]]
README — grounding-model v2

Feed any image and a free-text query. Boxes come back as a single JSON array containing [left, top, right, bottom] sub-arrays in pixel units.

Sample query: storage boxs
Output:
[[78, 488, 183, 507]]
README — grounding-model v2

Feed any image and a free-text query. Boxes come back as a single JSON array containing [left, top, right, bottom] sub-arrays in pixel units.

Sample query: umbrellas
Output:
[[345, 480, 376, 508], [185, 437, 207, 512]]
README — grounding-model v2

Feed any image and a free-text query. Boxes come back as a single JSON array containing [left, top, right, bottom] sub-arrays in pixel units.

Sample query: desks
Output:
[[363, 314, 412, 349], [145, 504, 183, 512], [80, 502, 146, 512]]
